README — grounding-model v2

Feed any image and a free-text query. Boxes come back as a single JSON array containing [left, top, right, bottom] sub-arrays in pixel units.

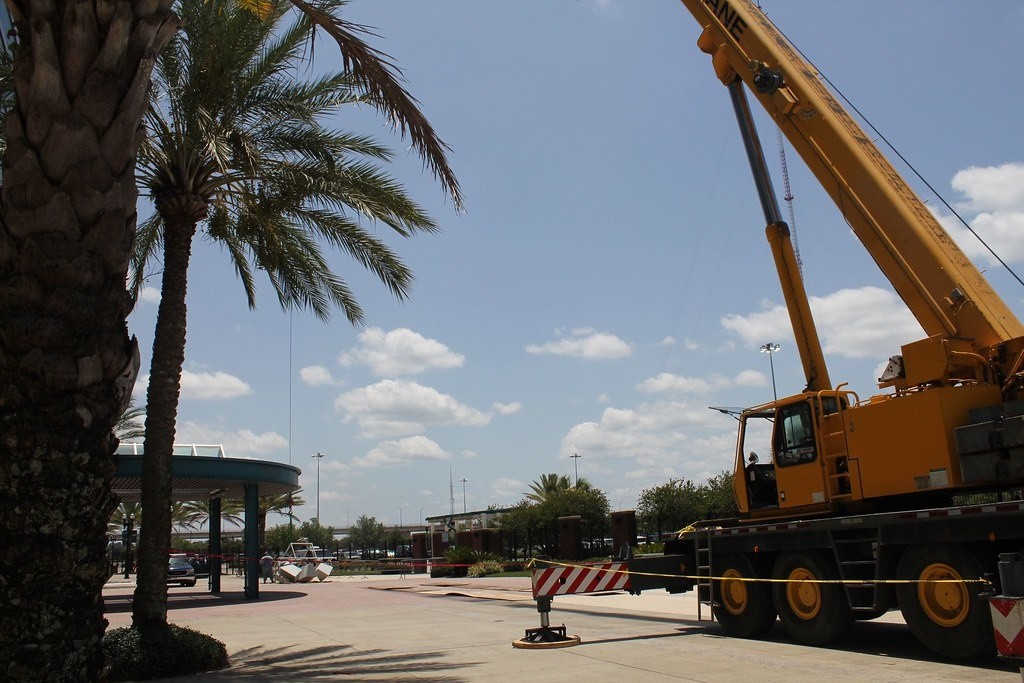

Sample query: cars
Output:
[[167, 555, 196, 587], [195, 545, 413, 566]]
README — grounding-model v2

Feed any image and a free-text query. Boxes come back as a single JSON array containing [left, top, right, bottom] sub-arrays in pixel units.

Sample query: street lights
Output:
[[759, 342, 782, 402], [459, 478, 469, 513], [569, 453, 581, 487], [312, 453, 327, 526]]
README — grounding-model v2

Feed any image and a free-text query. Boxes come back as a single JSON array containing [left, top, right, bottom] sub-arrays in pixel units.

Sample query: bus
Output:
[[296, 546, 328, 557]]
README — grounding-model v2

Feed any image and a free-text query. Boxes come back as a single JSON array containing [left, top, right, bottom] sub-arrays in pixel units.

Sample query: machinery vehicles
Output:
[[509, 1, 1024, 664]]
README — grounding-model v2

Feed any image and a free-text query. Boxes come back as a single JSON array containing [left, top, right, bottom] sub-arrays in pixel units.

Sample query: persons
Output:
[[259, 551, 276, 584]]
